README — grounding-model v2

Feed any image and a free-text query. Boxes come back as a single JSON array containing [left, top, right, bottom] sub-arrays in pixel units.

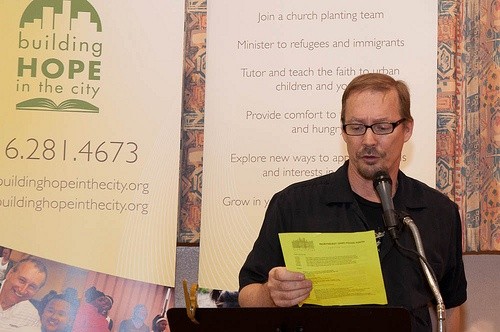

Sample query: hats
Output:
[[85, 286, 105, 303]]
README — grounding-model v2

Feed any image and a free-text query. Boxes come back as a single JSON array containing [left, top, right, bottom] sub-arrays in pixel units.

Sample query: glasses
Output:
[[342, 117, 405, 136]]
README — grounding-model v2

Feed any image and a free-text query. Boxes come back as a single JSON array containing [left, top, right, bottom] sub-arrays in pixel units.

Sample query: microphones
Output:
[[373, 170, 399, 241]]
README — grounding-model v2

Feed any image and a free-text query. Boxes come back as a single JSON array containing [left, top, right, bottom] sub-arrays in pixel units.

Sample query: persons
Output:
[[237, 73, 468, 332], [1, 258, 166, 331]]
